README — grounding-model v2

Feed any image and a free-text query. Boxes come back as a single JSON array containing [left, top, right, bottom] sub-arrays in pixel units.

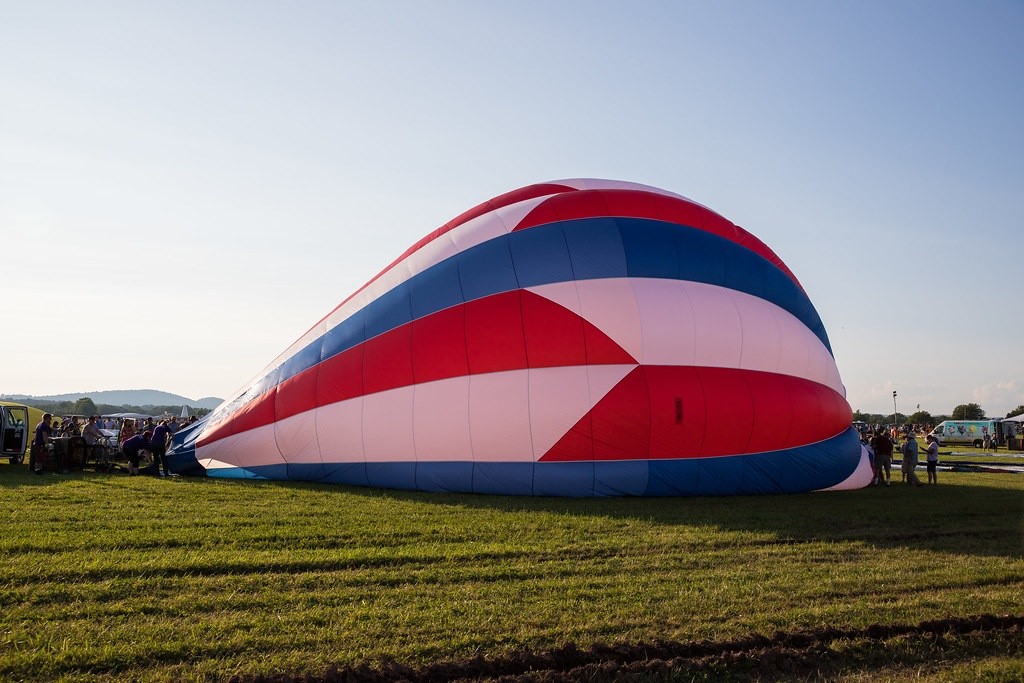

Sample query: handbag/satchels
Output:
[[903, 460, 913, 473]]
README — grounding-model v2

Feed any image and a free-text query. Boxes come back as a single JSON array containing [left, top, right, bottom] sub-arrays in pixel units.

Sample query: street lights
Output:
[[892, 390, 898, 424]]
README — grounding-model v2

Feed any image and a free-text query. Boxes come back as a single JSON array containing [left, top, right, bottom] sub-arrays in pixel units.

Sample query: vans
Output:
[[924, 419, 1003, 449], [0, 404, 30, 466]]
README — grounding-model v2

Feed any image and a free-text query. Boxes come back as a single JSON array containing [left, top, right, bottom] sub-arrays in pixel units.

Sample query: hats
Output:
[[985, 431, 987, 433], [189, 416, 198, 421], [907, 432, 915, 438], [89, 416, 95, 420]]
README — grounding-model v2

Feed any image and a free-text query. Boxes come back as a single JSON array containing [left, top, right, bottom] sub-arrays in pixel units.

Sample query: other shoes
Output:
[[35, 469, 42, 474], [886, 483, 890, 486], [130, 472, 140, 476], [874, 482, 877, 484]]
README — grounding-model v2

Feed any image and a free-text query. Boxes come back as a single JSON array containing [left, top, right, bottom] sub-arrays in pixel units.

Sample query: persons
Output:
[[919, 435, 940, 485], [895, 437, 914, 483], [982, 430, 990, 452], [902, 432, 923, 486], [30, 413, 197, 473], [857, 422, 935, 446], [872, 428, 894, 486], [991, 433, 999, 453]]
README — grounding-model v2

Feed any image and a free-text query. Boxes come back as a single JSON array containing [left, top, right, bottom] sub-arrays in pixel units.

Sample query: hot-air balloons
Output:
[[156, 178, 876, 494]]
